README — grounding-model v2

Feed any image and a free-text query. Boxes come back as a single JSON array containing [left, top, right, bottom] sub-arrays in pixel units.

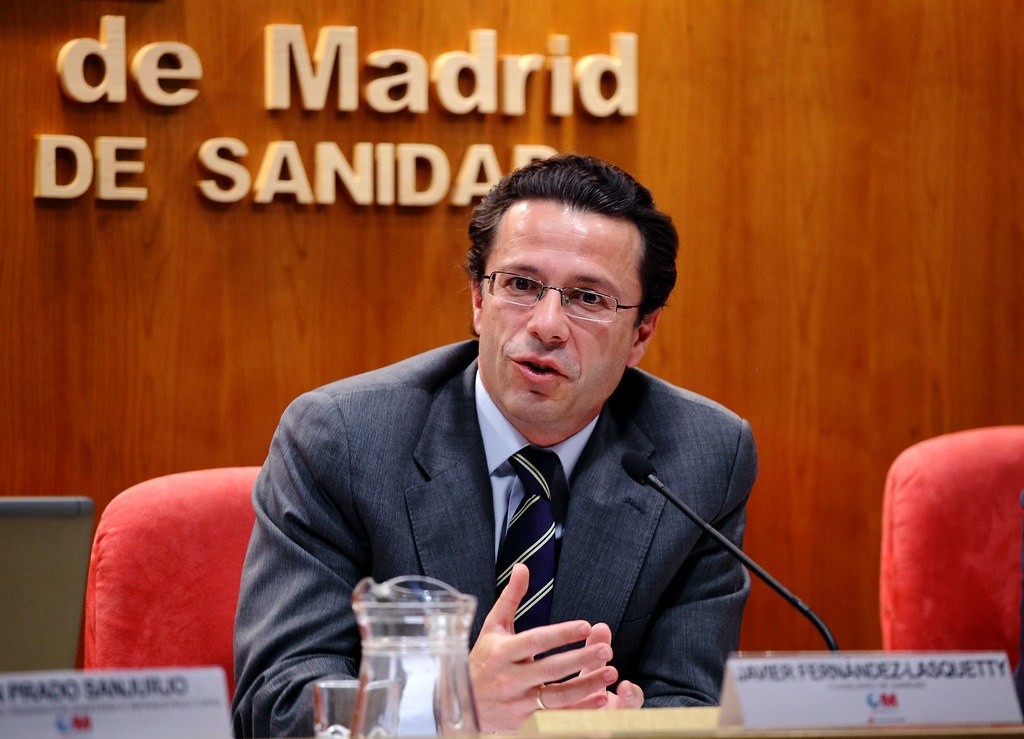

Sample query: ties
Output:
[[496, 445, 564, 658]]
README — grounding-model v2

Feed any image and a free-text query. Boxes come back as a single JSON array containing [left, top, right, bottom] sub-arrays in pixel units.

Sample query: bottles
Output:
[[351, 575, 476, 739]]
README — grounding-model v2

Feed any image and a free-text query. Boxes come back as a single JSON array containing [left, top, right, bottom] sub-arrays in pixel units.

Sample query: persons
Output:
[[229, 153, 758, 739]]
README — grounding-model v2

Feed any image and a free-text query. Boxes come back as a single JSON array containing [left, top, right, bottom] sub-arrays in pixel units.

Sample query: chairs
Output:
[[881, 425, 1024, 674], [84, 467, 267, 699]]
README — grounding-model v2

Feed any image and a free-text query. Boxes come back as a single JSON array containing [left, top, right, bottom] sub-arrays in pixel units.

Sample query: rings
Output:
[[536, 685, 549, 709]]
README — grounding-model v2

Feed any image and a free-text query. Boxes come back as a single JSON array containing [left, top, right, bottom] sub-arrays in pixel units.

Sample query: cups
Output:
[[315, 679, 398, 739]]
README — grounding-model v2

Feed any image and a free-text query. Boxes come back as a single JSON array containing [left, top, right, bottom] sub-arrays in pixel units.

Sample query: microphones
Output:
[[621, 452, 838, 650]]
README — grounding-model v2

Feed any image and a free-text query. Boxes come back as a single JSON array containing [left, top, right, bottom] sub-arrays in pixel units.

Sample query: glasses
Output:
[[481, 271, 643, 323]]
